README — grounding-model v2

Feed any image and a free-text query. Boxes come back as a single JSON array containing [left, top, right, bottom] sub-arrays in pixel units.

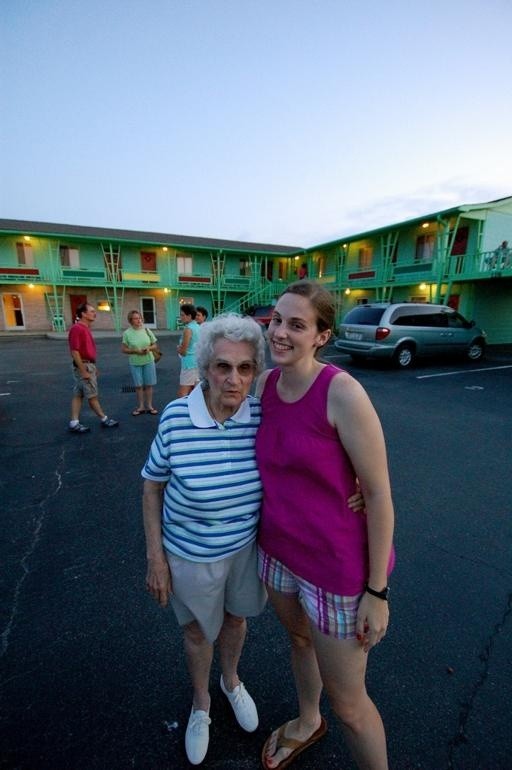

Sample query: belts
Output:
[[81, 360, 94, 363]]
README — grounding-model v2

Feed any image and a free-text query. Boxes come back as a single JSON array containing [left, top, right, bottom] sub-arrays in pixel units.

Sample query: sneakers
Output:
[[184, 694, 212, 764], [100, 417, 119, 427], [221, 672, 259, 733], [67, 424, 91, 433]]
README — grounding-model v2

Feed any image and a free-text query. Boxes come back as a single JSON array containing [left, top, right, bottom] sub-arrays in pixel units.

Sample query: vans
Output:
[[334, 302, 487, 369]]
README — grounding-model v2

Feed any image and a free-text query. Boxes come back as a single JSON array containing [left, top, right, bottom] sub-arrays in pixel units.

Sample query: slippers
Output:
[[146, 409, 158, 415], [131, 407, 145, 416], [261, 716, 327, 770]]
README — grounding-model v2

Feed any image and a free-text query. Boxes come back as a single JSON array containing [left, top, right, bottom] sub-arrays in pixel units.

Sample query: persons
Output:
[[176, 304, 201, 397], [484, 238, 511, 269], [64, 303, 119, 434], [194, 307, 208, 326], [139, 312, 367, 766], [254, 280, 396, 769], [120, 310, 158, 417]]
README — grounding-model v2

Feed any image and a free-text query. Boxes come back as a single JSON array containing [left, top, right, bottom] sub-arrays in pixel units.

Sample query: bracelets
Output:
[[364, 583, 391, 600]]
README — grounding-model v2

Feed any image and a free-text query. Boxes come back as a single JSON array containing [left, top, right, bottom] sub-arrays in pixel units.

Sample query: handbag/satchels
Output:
[[152, 350, 162, 364]]
